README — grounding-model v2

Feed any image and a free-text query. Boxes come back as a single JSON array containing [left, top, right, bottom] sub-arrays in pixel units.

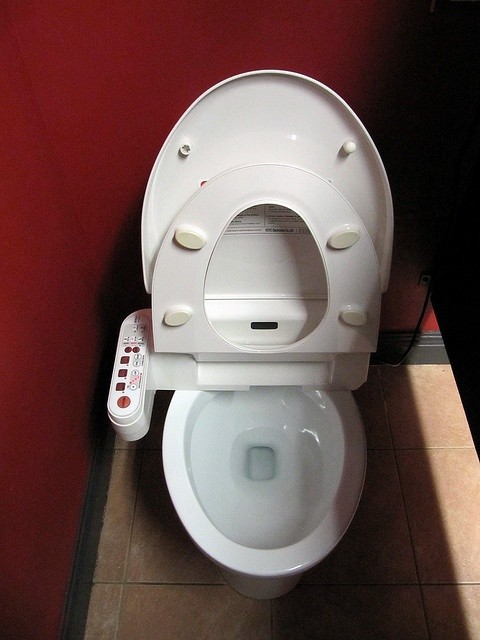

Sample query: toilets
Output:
[[106, 69, 394, 601]]
